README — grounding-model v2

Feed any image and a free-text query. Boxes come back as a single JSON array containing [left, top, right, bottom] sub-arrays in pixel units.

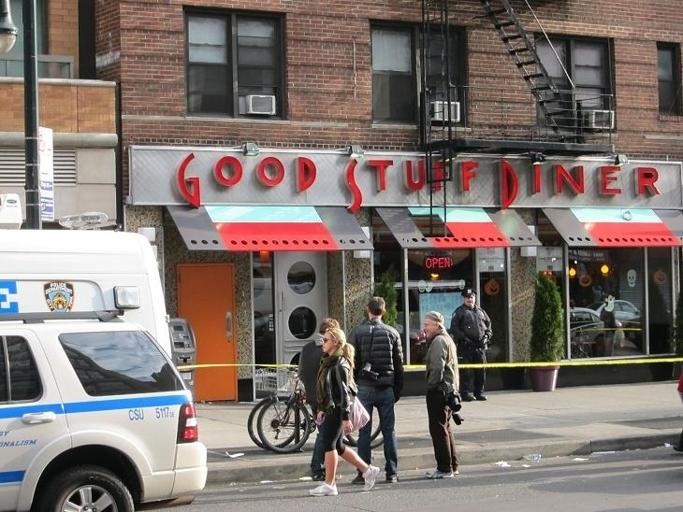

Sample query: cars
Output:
[[252, 262, 316, 327], [388, 321, 428, 365], [582, 300, 638, 332], [623, 308, 642, 350], [558, 308, 625, 354]]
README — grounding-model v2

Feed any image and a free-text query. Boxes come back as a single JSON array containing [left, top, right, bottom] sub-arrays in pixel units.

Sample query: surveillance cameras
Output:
[[0, 0, 19, 52]]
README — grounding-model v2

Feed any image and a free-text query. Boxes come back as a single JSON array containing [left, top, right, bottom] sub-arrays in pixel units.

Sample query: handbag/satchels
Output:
[[344, 393, 370, 435]]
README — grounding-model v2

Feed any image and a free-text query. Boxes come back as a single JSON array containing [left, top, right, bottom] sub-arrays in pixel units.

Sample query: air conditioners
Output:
[[581, 108, 614, 129], [244, 93, 278, 116], [429, 101, 460, 123]]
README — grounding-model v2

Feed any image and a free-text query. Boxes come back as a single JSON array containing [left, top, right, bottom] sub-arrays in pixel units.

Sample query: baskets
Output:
[[256, 366, 294, 394]]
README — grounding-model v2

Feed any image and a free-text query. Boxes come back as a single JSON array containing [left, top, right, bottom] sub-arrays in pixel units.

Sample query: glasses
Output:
[[322, 338, 334, 343]]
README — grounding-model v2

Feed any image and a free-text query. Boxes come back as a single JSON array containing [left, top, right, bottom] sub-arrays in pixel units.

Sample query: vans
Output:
[[0, 226, 171, 389]]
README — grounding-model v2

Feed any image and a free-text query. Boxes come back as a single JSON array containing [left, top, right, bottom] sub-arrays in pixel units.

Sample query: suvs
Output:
[[0, 307, 209, 512]]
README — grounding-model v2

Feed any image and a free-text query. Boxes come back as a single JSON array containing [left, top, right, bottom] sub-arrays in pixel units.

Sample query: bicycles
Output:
[[246, 365, 382, 452], [256, 374, 388, 454]]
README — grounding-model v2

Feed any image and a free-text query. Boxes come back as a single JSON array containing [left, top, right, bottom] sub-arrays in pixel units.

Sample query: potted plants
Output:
[[526, 270, 567, 391]]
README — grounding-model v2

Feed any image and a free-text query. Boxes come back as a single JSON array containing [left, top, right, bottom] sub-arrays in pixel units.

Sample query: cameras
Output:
[[447, 391, 462, 412], [361, 362, 379, 381]]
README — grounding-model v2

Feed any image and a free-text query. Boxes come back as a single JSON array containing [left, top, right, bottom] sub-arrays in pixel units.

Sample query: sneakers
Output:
[[310, 482, 338, 497], [425, 469, 454, 478], [453, 469, 459, 475], [362, 464, 380, 490], [299, 468, 326, 481]]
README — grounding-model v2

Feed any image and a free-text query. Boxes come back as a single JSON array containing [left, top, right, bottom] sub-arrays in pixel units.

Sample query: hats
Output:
[[460, 287, 477, 296], [426, 309, 443, 329]]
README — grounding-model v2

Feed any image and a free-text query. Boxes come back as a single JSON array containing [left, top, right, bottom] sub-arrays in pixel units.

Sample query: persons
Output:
[[297, 317, 341, 482], [600, 299, 616, 356], [346, 296, 404, 484], [308, 327, 381, 497], [451, 286, 493, 401], [423, 311, 462, 479]]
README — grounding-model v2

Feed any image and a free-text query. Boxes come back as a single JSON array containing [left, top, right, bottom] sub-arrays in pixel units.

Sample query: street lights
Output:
[[0, 1, 39, 229]]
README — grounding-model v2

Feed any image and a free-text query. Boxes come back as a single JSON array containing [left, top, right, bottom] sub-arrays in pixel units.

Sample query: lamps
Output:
[[520, 152, 547, 166], [336, 145, 366, 160], [603, 153, 629, 168], [518, 225, 536, 258], [350, 226, 371, 260], [233, 143, 260, 156]]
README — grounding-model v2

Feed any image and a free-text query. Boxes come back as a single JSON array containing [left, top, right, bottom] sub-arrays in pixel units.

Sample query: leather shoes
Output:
[[385, 473, 399, 483], [473, 393, 487, 400], [462, 393, 476, 401], [352, 474, 365, 484]]
